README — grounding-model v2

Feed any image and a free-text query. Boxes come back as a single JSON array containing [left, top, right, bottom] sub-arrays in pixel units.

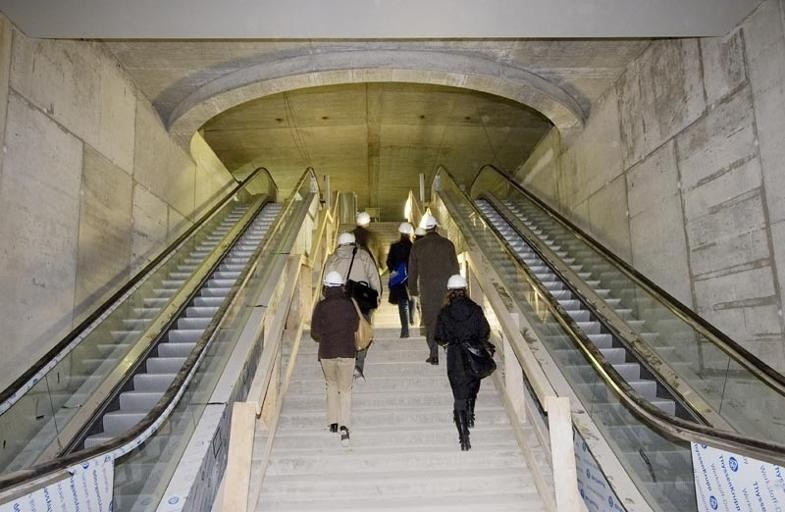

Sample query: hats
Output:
[[322, 270, 346, 290], [419, 213, 437, 232], [398, 222, 427, 236], [447, 275, 469, 291], [338, 231, 356, 246], [355, 212, 371, 228]]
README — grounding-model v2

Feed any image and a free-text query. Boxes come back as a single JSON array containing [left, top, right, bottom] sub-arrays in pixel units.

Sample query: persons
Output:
[[408, 213, 460, 365], [319, 232, 383, 384], [385, 222, 415, 338], [434, 274, 496, 451], [349, 212, 387, 297], [310, 271, 361, 446]]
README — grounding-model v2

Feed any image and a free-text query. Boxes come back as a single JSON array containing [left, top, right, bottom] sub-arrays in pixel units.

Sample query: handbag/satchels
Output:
[[459, 338, 498, 380], [351, 296, 375, 353], [386, 261, 410, 289], [344, 277, 379, 310]]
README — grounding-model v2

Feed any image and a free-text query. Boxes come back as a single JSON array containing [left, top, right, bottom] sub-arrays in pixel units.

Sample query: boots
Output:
[[465, 392, 478, 429], [453, 408, 472, 452], [408, 300, 417, 326], [398, 302, 410, 339]]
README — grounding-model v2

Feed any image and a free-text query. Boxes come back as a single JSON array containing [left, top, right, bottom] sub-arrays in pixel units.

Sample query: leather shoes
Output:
[[425, 356, 439, 366]]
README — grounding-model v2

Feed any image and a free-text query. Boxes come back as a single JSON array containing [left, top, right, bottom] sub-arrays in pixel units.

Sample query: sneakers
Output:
[[352, 365, 367, 386]]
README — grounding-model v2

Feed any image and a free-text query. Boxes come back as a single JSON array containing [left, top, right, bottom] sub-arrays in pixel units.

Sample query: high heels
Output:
[[330, 422, 338, 433], [340, 424, 352, 448]]
